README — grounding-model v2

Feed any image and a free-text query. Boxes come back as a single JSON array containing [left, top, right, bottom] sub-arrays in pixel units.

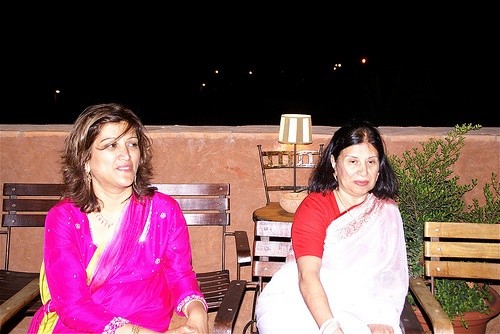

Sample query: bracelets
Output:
[[320, 319, 338, 334], [132, 324, 139, 334]]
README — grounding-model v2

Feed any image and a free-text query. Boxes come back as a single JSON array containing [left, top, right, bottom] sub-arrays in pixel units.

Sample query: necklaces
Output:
[[335, 187, 373, 224], [94, 208, 114, 229]]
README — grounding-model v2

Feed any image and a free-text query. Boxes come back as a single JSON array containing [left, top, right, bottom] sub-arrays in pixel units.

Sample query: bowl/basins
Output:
[[279, 193, 307, 214]]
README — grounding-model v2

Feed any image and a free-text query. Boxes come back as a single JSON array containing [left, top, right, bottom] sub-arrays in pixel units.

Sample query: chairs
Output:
[[214, 221, 430, 334], [409, 221, 500, 334], [1, 182, 251, 315], [251, 144, 325, 275]]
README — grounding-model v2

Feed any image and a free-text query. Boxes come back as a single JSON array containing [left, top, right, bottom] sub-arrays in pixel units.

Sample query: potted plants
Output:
[[390, 125, 500, 334]]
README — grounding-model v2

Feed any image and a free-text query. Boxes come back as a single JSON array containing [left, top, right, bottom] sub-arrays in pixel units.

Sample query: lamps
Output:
[[277, 113, 311, 214]]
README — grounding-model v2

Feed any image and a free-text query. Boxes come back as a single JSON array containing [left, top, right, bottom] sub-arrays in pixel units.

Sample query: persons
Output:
[[255, 124, 410, 334], [28, 103, 208, 334]]
